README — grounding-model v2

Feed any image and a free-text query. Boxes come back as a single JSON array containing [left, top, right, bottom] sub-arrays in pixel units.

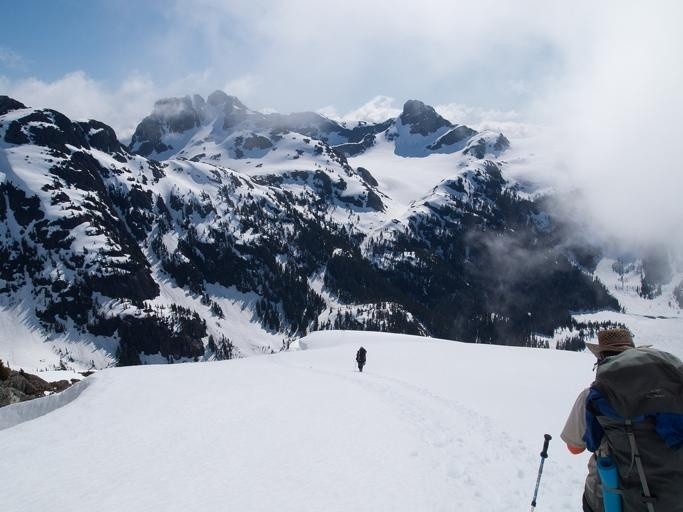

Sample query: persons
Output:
[[356, 346, 367, 372], [560, 329, 683, 512]]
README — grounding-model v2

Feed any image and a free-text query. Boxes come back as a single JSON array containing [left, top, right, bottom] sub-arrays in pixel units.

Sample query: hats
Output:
[[585, 329, 653, 358]]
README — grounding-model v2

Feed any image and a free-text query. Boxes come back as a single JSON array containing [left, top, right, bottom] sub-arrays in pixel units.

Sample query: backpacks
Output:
[[592, 348, 683, 512]]
